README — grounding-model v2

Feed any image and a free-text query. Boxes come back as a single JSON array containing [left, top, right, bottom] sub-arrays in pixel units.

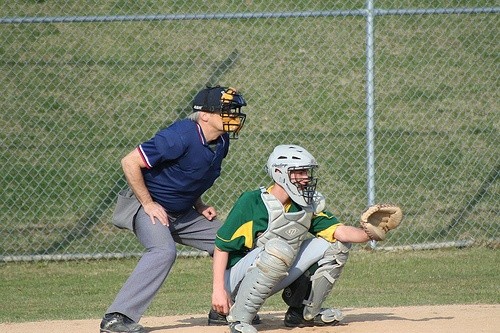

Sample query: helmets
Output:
[[185, 85, 248, 139], [266, 144, 319, 207]]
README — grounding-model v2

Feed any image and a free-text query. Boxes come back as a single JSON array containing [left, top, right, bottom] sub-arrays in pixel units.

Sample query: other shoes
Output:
[[284, 305, 344, 327]]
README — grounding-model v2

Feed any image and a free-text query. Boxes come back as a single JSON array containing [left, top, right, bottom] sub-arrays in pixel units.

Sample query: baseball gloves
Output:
[[358, 203, 402, 242]]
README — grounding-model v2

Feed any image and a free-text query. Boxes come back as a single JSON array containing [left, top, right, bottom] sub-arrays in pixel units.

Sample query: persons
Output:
[[212, 144, 403, 333], [99, 85, 260, 333]]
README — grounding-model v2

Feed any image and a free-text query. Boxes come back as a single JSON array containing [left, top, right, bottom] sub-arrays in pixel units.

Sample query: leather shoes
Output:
[[100, 312, 145, 333], [208, 308, 262, 326]]
